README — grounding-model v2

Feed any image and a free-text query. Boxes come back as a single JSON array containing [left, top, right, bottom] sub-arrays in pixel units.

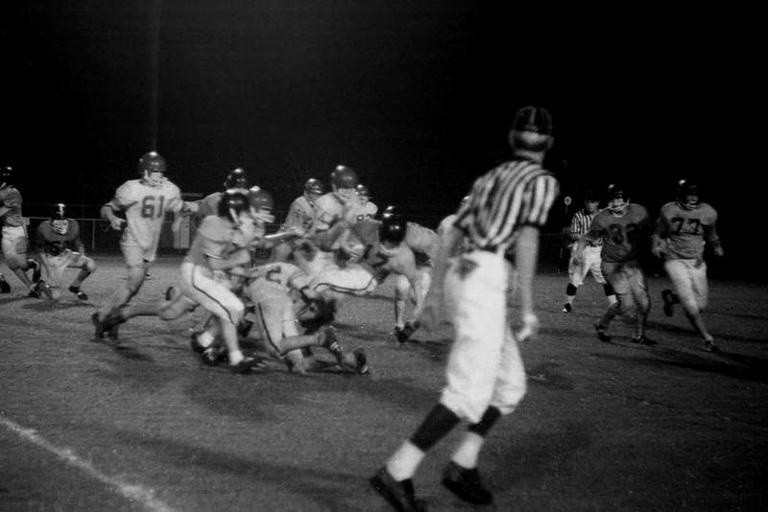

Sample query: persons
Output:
[[29, 203, 96, 301], [0, 165, 41, 294], [370, 105, 562, 512], [93, 149, 471, 375], [651, 178, 724, 353], [573, 184, 658, 346], [563, 193, 618, 312]]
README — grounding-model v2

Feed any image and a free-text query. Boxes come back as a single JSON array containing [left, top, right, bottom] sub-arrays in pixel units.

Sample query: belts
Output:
[[483, 245, 515, 264]]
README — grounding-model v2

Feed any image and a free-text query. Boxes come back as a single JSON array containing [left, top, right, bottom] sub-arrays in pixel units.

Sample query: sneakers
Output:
[[370, 465, 415, 512], [69, 285, 88, 300], [663, 289, 675, 316], [395, 319, 420, 342], [91, 304, 129, 341], [632, 335, 657, 345], [28, 259, 53, 299], [190, 303, 369, 373], [441, 461, 492, 504], [593, 319, 611, 342], [705, 340, 721, 352]]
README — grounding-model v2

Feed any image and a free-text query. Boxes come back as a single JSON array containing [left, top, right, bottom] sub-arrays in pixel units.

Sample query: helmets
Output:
[[220, 169, 273, 218], [54, 204, 67, 215], [303, 163, 370, 197], [512, 107, 552, 134], [381, 204, 406, 240], [138, 150, 167, 176], [608, 184, 627, 197], [678, 178, 698, 195]]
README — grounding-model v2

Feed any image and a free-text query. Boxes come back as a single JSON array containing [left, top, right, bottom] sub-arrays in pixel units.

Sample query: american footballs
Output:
[[299, 308, 336, 327]]
